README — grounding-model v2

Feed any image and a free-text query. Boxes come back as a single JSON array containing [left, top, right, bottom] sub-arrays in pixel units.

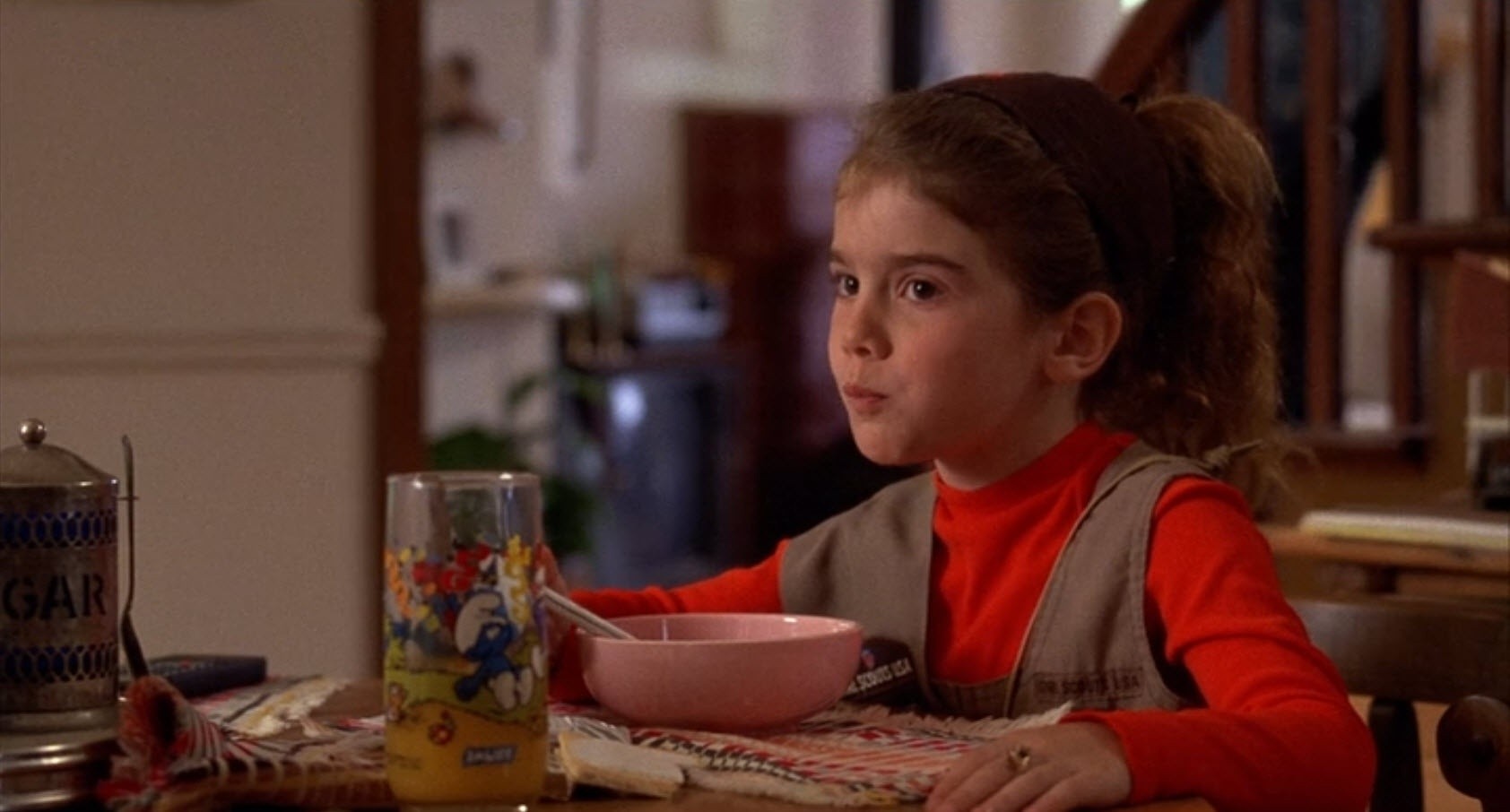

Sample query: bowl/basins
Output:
[[575, 614, 866, 739]]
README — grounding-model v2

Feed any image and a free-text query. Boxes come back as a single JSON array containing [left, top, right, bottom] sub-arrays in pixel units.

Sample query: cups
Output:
[[0, 417, 120, 812], [382, 470, 548, 812]]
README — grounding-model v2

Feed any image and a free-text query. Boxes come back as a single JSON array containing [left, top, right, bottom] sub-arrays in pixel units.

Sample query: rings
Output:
[[1009, 745, 1032, 772]]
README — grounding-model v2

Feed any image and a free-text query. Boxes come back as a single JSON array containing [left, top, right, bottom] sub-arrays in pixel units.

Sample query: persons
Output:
[[541, 72, 1374, 812]]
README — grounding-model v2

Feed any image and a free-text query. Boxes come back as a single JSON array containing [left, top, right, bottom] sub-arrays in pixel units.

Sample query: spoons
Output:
[[123, 435, 149, 682]]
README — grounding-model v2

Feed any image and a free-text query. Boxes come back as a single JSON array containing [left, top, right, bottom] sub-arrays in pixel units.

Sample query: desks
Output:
[[187, 676, 1216, 812]]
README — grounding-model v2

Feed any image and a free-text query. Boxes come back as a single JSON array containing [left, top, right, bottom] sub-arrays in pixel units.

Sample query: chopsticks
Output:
[[540, 584, 633, 639]]
